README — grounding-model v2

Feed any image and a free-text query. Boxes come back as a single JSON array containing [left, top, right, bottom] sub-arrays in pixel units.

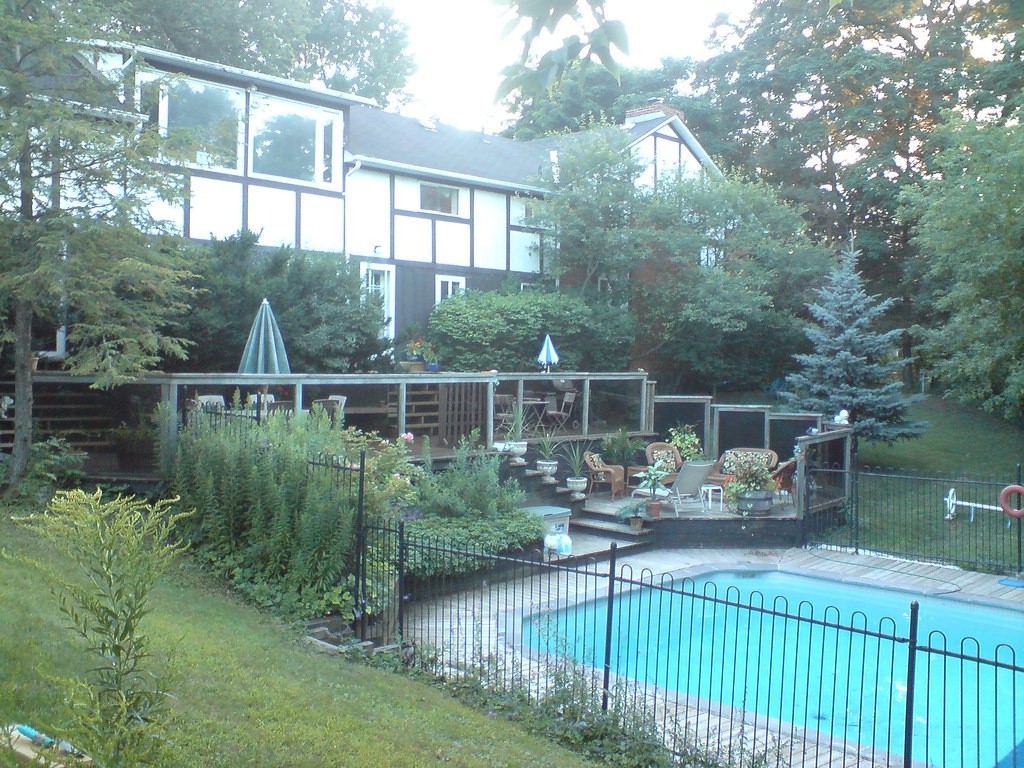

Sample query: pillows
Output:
[[651, 450, 676, 473], [720, 451, 773, 475], [590, 453, 605, 480]]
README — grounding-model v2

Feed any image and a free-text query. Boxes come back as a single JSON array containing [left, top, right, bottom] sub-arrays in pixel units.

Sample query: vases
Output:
[[736, 491, 773, 517], [406, 353, 421, 362]]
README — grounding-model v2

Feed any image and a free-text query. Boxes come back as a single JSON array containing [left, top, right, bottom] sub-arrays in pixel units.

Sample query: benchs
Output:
[[711, 448, 778, 486]]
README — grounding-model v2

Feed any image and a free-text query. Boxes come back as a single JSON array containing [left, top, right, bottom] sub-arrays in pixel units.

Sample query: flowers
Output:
[[723, 477, 783, 537], [405, 338, 438, 364]]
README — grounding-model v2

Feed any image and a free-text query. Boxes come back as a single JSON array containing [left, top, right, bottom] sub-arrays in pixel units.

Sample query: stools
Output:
[[701, 484, 724, 512]]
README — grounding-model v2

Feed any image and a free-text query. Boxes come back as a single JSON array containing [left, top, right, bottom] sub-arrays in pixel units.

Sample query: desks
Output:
[[626, 466, 648, 497], [514, 401, 549, 438]]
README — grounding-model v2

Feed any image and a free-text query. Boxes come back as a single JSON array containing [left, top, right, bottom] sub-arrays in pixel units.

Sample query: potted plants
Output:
[[633, 459, 681, 517], [532, 424, 566, 483], [31, 350, 42, 369], [504, 397, 535, 464], [615, 498, 652, 530], [109, 418, 158, 471], [555, 438, 594, 499]]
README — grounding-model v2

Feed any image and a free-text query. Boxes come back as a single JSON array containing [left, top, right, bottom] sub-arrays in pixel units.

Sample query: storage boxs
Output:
[[521, 506, 571, 541]]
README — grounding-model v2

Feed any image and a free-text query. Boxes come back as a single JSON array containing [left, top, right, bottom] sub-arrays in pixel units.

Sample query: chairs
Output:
[[770, 460, 798, 511], [583, 451, 624, 503], [546, 392, 576, 433], [329, 396, 347, 411], [247, 394, 275, 410], [493, 395, 514, 431], [645, 442, 682, 485], [198, 395, 225, 411], [267, 400, 295, 419], [313, 400, 341, 433], [631, 461, 713, 517]]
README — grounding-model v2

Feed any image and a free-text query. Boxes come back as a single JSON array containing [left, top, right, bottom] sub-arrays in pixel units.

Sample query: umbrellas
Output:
[[536, 334, 559, 374], [238, 298, 290, 412]]
